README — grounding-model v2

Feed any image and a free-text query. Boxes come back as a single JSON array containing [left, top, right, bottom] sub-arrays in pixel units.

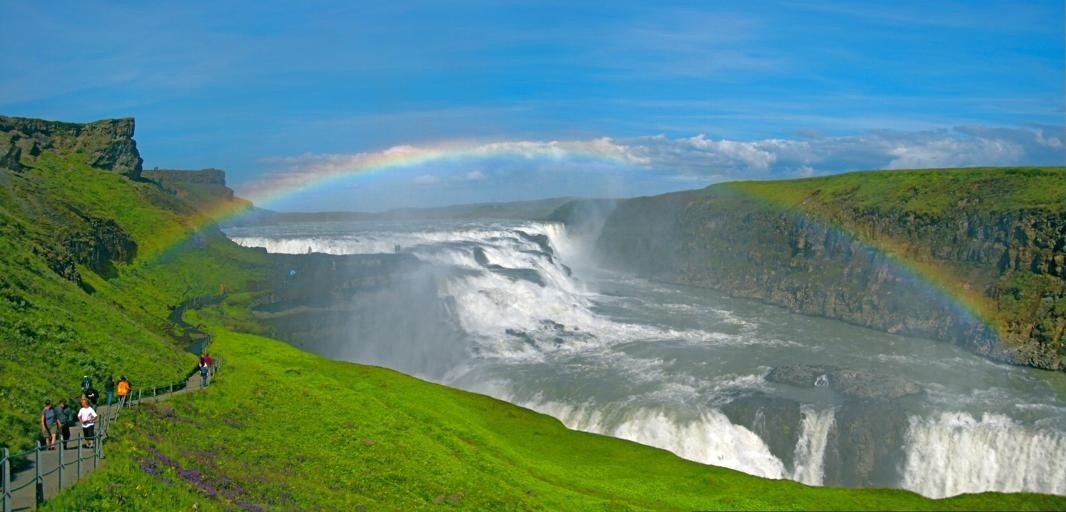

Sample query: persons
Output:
[[42, 373, 133, 450], [198, 353, 212, 386]]
